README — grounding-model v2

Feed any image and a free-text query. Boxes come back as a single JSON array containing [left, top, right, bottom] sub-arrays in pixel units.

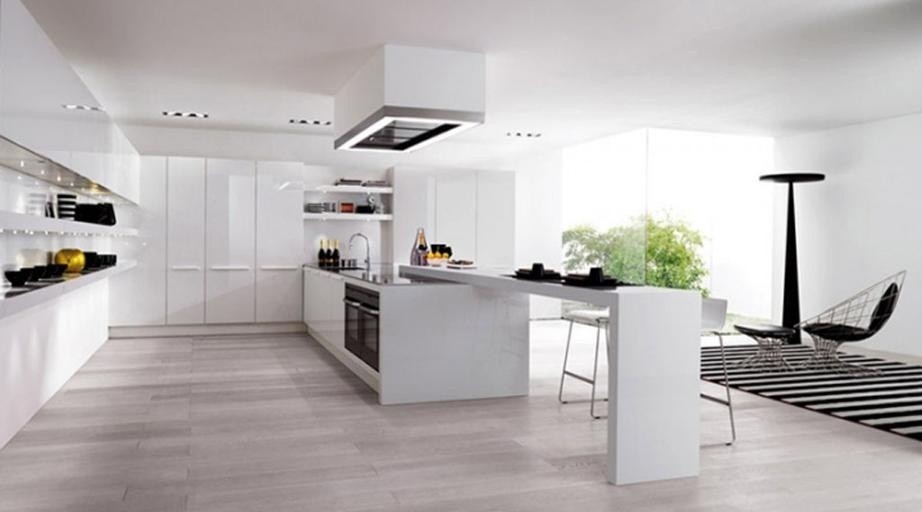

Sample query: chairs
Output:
[[558, 304, 610, 421], [794, 269, 907, 378], [702, 295, 737, 447]]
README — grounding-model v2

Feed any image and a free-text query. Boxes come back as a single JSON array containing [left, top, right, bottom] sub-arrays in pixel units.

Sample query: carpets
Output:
[[702, 342, 922, 443]]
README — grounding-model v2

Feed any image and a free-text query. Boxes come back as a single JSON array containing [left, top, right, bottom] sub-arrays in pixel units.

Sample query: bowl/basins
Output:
[[357, 205, 376, 214], [77, 204, 110, 223], [5, 263, 68, 287], [98, 255, 116, 267], [83, 252, 101, 268], [427, 258, 448, 266]]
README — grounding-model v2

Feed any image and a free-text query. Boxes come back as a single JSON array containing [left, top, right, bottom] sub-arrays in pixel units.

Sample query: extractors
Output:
[[333, 45, 489, 155]]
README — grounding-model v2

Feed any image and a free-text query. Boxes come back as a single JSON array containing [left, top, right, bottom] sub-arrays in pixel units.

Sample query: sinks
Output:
[[321, 265, 366, 272], [348, 232, 371, 271]]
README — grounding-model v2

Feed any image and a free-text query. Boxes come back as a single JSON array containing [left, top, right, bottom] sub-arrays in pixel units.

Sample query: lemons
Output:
[[427, 251, 449, 259]]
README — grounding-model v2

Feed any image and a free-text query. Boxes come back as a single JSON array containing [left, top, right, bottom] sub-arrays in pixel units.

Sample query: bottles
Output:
[[410, 227, 430, 264], [317, 240, 340, 267]]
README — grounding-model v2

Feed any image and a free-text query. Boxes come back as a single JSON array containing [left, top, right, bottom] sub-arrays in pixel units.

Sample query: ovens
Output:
[[343, 282, 380, 372]]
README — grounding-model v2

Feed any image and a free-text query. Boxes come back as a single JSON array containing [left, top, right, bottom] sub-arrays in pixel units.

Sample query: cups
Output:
[[58, 194, 77, 221], [341, 255, 358, 267], [431, 243, 446, 254], [25, 192, 46, 216], [533, 264, 544, 279], [588, 268, 604, 287]]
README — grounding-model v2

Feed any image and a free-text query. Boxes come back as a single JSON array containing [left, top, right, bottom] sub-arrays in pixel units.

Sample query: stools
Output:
[[734, 321, 797, 373]]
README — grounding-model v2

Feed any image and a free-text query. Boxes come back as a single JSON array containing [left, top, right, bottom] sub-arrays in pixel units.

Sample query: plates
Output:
[[0, 286, 29, 293], [515, 268, 561, 281], [448, 264, 479, 269], [562, 273, 617, 289], [308, 203, 325, 213]]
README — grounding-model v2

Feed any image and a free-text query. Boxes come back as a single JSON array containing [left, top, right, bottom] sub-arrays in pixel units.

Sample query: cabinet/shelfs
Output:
[[0, 210, 140, 318], [108, 154, 516, 338]]
